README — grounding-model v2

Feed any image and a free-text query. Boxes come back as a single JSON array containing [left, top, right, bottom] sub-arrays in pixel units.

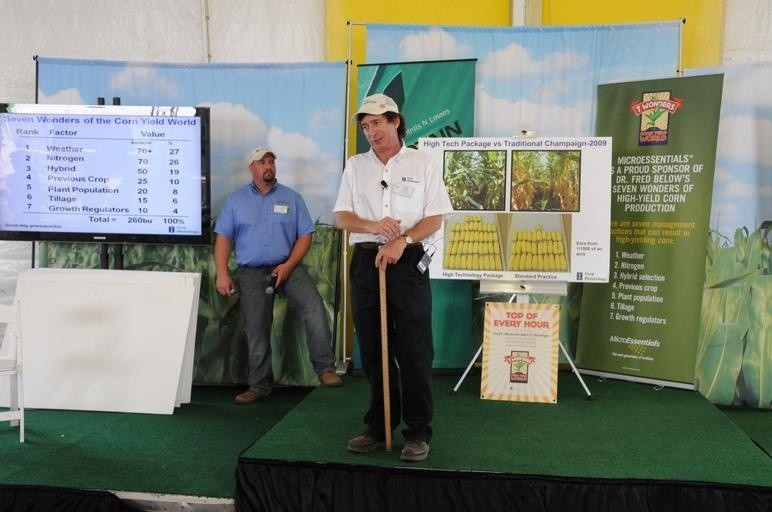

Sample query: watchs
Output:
[[403, 233, 413, 245]]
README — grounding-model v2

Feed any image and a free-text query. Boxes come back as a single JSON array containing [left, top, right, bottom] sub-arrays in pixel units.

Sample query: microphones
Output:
[[265, 277, 278, 297]]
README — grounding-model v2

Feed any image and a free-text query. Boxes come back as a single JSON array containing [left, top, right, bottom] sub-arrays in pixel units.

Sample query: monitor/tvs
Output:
[[0, 102, 213, 246]]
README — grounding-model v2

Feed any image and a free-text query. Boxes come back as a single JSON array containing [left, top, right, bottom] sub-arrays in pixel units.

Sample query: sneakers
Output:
[[234, 387, 263, 404], [317, 368, 343, 388]]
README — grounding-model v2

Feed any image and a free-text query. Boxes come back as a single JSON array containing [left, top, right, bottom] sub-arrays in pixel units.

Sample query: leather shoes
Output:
[[347, 433, 394, 453], [400, 438, 430, 462]]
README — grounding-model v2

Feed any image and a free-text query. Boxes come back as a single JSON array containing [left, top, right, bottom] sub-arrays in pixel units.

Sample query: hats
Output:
[[351, 93, 399, 123], [248, 146, 276, 166]]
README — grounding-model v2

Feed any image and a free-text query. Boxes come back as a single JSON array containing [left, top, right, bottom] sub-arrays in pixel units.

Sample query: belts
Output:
[[354, 241, 423, 250]]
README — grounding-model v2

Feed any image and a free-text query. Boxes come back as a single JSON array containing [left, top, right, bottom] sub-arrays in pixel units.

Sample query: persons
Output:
[[332, 94, 455, 461], [215, 148, 344, 403]]
